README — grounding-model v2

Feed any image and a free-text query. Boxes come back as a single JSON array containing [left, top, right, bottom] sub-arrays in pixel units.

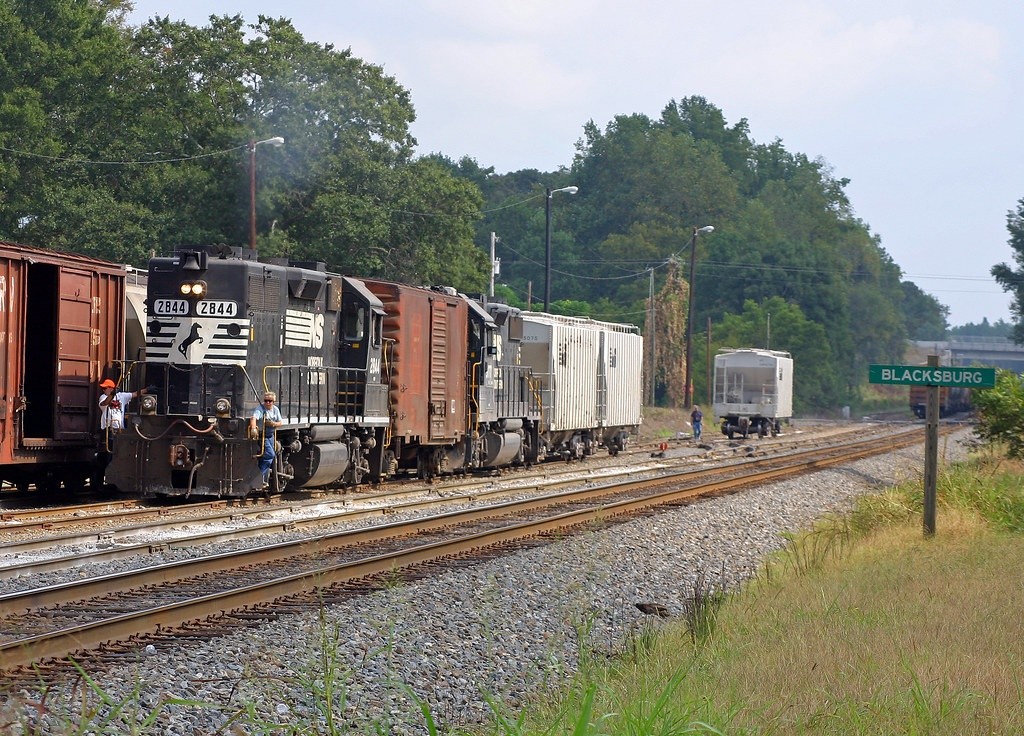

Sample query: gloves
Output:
[[251, 429, 258, 438], [266, 421, 274, 426], [110, 387, 120, 395], [146, 385, 159, 394]]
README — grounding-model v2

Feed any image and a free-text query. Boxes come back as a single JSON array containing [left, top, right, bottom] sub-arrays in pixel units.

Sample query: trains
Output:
[[1, 237, 163, 500], [101, 249, 643, 500], [909, 358, 985, 419], [714, 346, 794, 438]]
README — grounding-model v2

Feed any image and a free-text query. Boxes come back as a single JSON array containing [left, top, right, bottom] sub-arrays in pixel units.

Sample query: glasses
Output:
[[264, 399, 273, 403]]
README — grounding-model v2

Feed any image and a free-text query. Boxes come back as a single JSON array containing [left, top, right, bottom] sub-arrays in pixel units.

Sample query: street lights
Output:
[[682, 224, 717, 408], [544, 185, 579, 313], [248, 137, 287, 251]]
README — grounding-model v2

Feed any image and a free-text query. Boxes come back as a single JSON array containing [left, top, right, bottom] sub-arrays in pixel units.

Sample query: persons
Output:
[[690, 405, 703, 440], [99, 380, 157, 448], [250, 392, 282, 491]]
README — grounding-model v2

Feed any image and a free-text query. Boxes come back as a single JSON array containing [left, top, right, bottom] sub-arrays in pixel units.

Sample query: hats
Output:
[[99, 379, 115, 388]]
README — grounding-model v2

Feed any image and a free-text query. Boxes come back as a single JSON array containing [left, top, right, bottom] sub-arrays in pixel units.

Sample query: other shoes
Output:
[[262, 482, 269, 488]]
